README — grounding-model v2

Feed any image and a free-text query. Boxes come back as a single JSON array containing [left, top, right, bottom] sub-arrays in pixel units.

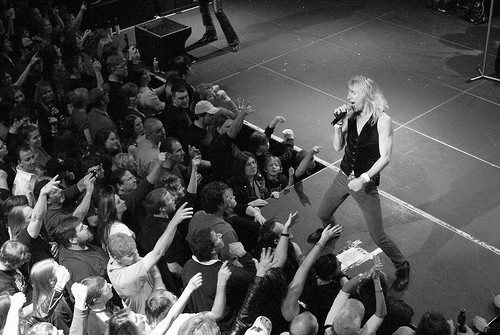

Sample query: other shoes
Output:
[[393, 261, 411, 291], [306, 226, 339, 245], [197, 33, 217, 46], [229, 39, 240, 52]]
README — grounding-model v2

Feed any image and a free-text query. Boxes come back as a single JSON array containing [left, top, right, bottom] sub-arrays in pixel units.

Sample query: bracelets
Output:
[[484, 327, 488, 332], [281, 233, 290, 237], [376, 288, 383, 292], [55, 284, 64, 291], [334, 121, 343, 129], [253, 213, 260, 216], [361, 172, 370, 183]]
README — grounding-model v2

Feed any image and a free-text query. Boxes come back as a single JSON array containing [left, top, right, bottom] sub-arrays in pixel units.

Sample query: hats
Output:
[[194, 99, 220, 116], [89, 83, 111, 103]]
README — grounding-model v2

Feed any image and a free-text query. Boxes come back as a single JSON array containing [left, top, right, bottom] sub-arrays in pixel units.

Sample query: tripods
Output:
[[466, 0, 500, 84]]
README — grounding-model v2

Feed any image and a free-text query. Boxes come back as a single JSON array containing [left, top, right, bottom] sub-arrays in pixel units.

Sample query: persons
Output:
[[307, 75, 411, 289], [0, 55, 342, 335], [0, 0, 140, 83], [306, 254, 500, 335], [199, 0, 240, 52]]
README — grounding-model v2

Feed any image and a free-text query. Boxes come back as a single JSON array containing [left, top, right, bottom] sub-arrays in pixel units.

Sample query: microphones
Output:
[[331, 103, 353, 126]]
